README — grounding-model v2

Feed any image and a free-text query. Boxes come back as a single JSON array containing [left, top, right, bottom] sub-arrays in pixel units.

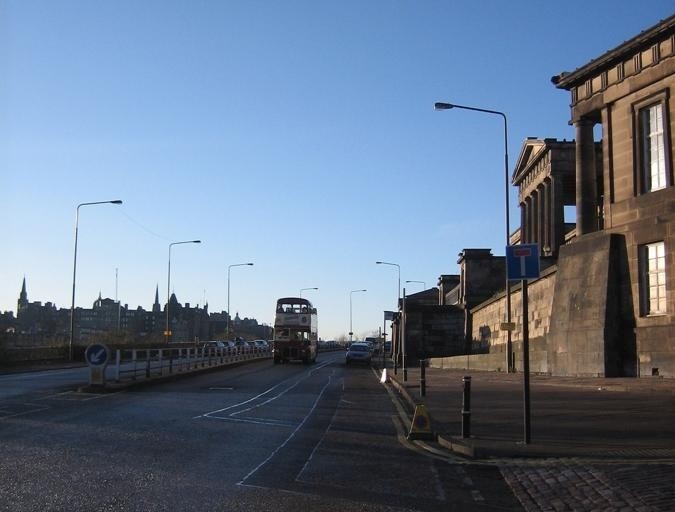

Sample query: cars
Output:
[[346, 341, 375, 365], [193, 340, 273, 357]]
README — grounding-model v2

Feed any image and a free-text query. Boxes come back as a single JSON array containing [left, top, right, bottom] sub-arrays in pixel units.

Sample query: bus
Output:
[[273, 298, 318, 364], [366, 338, 384, 351]]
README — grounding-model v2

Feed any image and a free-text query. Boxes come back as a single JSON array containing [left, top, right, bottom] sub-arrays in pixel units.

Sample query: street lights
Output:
[[165, 241, 201, 345], [350, 290, 367, 344], [434, 103, 512, 373], [227, 263, 253, 340], [69, 200, 123, 361], [376, 261, 426, 314], [300, 288, 318, 313]]
